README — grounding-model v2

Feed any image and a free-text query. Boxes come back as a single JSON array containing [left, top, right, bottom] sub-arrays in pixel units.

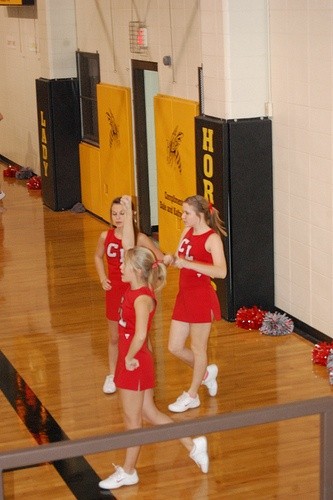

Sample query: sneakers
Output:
[[98, 463, 139, 489], [201, 364, 218, 397], [103, 375, 116, 394], [188, 436, 208, 473], [168, 391, 199, 413]]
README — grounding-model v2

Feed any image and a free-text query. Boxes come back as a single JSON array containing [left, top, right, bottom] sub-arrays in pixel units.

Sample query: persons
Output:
[[93, 198, 166, 394], [97, 194, 209, 489], [162, 196, 227, 411]]
